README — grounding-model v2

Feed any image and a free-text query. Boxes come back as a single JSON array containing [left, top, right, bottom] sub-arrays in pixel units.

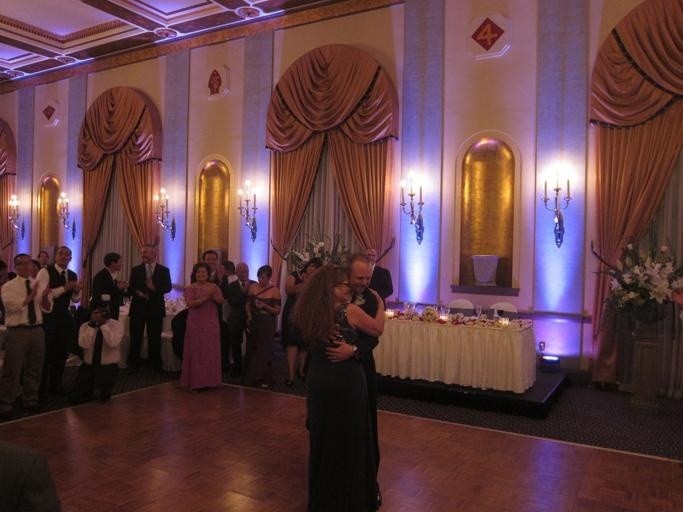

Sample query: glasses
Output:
[[336, 283, 350, 287]]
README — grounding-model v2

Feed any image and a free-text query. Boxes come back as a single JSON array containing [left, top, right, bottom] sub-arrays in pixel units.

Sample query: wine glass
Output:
[[400, 302, 449, 319], [475, 306, 481, 319]]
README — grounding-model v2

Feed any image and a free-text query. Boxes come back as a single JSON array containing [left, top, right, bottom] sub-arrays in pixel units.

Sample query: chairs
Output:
[[447, 299, 474, 317], [486, 301, 518, 322]]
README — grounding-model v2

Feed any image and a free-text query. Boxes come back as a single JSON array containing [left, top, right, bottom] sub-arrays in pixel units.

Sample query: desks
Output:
[[114, 300, 246, 372], [375, 310, 537, 393]]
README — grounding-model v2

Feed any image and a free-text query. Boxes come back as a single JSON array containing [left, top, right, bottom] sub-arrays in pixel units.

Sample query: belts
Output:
[[9, 325, 41, 330]]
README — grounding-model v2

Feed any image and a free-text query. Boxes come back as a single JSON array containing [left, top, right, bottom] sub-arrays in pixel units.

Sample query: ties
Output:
[[25, 280, 35, 323], [241, 282, 245, 288], [61, 271, 65, 287], [93, 331, 102, 366], [146, 264, 151, 279]]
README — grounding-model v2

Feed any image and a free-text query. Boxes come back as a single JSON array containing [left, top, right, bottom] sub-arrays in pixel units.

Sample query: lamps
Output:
[[56, 191, 75, 239], [538, 165, 572, 246], [7, 194, 25, 240], [151, 187, 176, 243], [236, 180, 260, 241], [398, 167, 431, 245], [540, 354, 560, 374]]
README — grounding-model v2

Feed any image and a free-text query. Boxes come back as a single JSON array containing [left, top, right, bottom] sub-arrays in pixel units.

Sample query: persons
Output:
[[75, 245, 174, 409], [285, 247, 393, 378], [319, 252, 389, 506], [180, 246, 282, 394], [284, 262, 387, 511], [1, 246, 84, 420], [1, 440, 63, 511]]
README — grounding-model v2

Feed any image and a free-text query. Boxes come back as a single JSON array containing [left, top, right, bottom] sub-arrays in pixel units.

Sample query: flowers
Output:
[[287, 231, 351, 270], [585, 211, 682, 344]]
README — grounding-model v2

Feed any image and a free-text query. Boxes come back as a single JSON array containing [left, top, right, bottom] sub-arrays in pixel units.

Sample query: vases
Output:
[[630, 319, 659, 340]]
